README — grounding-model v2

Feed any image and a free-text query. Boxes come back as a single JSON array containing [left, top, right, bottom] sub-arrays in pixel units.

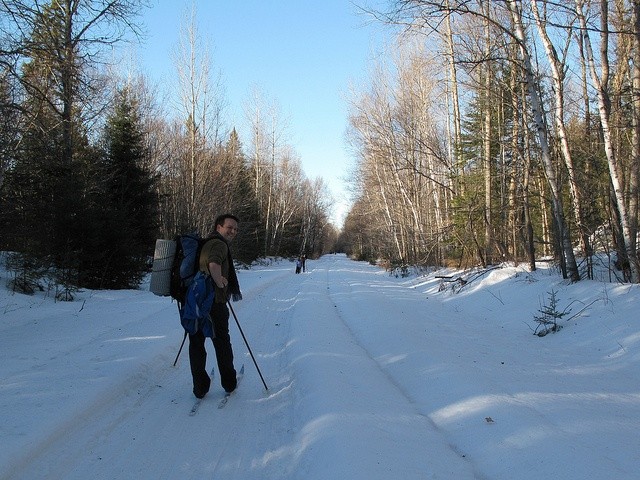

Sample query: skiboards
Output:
[[188, 364, 245, 416]]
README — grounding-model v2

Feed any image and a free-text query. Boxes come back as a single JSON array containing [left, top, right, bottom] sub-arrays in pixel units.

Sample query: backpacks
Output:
[[170, 232, 228, 302]]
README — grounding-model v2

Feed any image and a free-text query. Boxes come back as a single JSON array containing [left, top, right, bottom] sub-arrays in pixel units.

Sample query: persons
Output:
[[181, 213, 240, 398], [295, 251, 306, 274]]
